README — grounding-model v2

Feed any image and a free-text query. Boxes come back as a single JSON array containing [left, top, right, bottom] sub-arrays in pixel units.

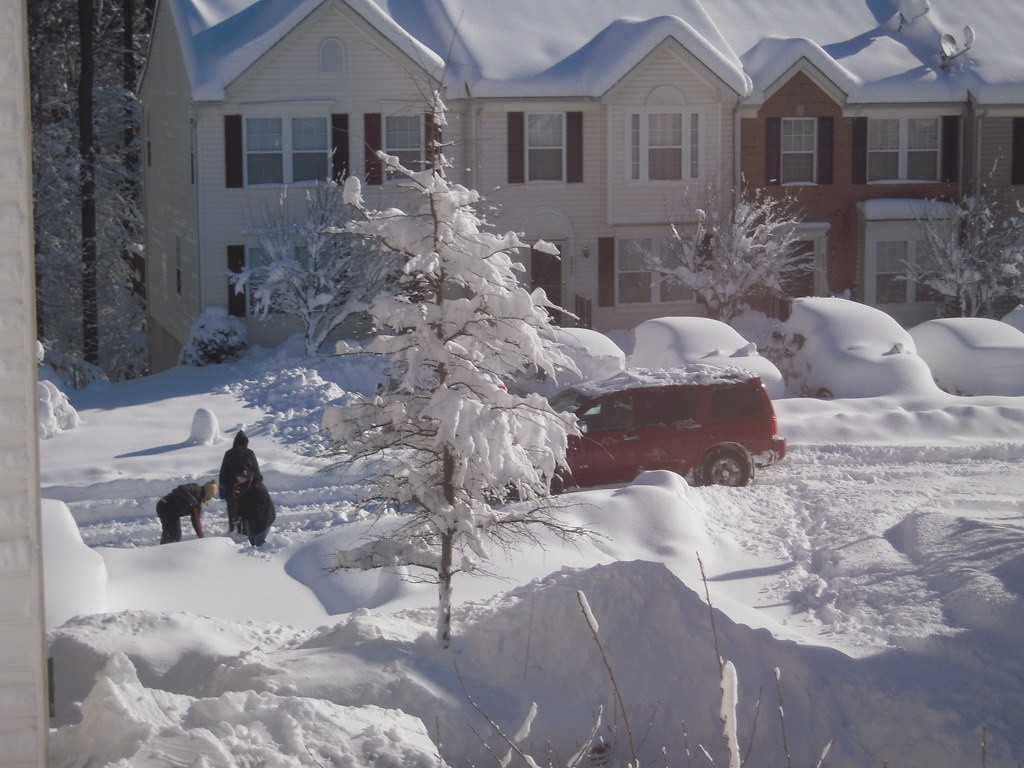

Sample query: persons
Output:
[[156, 480, 217, 545], [219, 430, 260, 532], [235, 463, 275, 547]]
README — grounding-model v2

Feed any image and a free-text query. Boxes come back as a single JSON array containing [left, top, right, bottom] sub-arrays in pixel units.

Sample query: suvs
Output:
[[547, 363, 787, 494]]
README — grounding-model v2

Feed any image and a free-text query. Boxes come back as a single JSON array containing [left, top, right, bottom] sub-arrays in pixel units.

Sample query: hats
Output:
[[204, 479, 218, 496]]
